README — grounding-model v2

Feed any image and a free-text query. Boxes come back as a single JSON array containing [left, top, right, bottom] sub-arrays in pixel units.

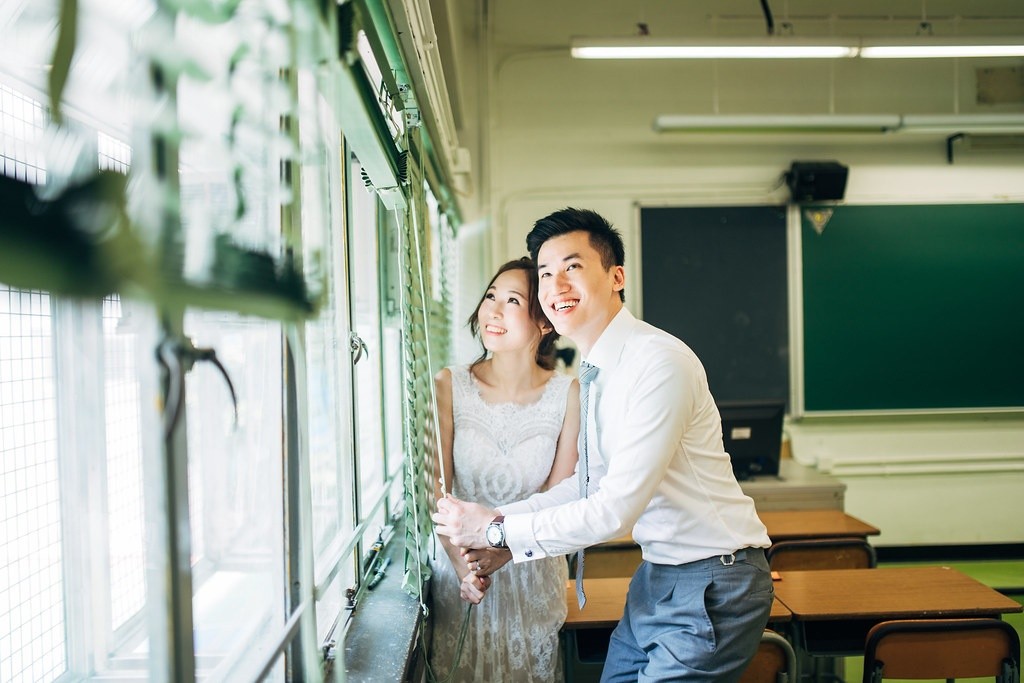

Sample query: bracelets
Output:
[[486, 516, 505, 547]]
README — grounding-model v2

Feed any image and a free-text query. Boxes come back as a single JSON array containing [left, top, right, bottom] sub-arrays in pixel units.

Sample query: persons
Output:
[[434, 257, 580, 683], [434, 209, 773, 683]]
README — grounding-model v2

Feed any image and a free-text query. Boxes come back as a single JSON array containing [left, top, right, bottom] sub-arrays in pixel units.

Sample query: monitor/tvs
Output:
[[714, 399, 785, 480]]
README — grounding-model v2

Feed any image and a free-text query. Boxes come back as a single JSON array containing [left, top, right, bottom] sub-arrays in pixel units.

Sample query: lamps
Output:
[[571, 0, 1024, 137]]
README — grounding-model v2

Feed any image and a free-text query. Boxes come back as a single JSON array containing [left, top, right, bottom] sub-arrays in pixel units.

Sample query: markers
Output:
[[368, 557, 391, 590]]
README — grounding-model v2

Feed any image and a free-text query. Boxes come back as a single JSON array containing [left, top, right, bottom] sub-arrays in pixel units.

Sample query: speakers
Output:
[[787, 161, 848, 201]]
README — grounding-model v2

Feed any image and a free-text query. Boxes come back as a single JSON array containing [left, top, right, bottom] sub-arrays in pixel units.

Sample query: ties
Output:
[[576, 361, 599, 611]]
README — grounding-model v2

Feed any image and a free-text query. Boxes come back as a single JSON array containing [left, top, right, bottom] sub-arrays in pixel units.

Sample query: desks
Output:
[[740, 458, 847, 511], [555, 471, 1024, 683]]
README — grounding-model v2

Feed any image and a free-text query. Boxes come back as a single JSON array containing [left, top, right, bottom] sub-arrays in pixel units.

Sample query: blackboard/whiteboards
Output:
[[633, 198, 1024, 421]]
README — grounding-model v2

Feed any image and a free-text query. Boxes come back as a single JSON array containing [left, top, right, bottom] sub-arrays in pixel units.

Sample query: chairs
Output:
[[767, 539, 876, 571], [862, 618, 1024, 683]]
